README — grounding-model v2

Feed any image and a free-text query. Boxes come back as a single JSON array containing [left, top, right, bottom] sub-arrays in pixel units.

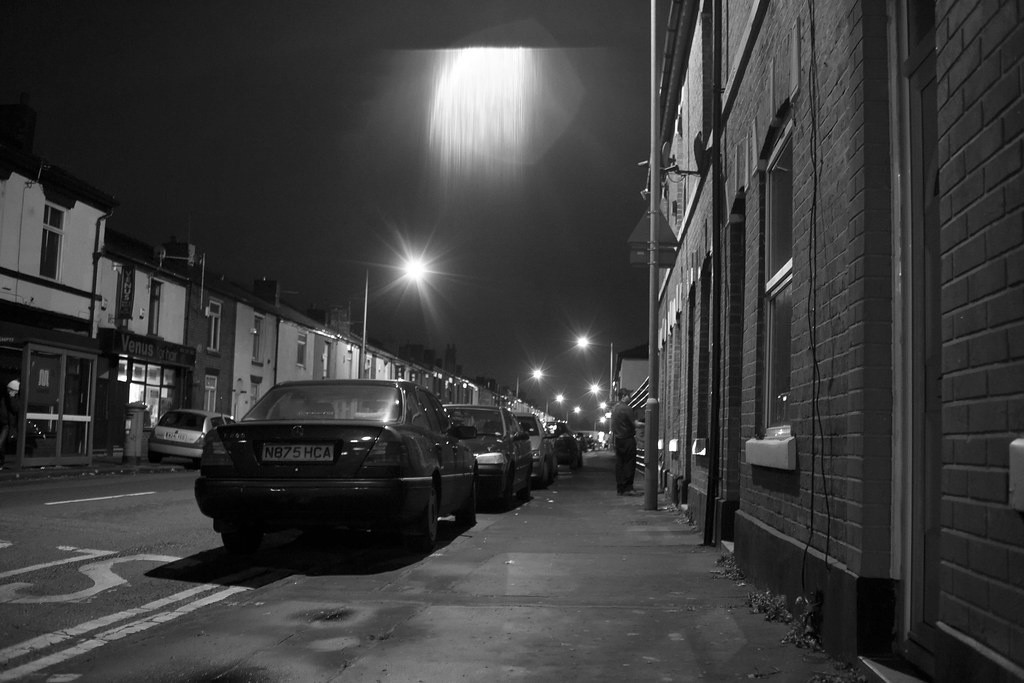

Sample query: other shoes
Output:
[[620, 489, 644, 496], [0, 464, 5, 471]]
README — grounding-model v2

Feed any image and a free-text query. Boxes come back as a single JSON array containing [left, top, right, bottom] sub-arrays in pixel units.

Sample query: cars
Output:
[[148, 408, 236, 468], [510, 412, 595, 489], [442, 404, 532, 506], [195, 380, 479, 554]]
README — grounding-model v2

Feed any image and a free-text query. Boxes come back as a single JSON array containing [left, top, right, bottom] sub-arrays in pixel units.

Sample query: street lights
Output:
[[516, 369, 543, 399], [361, 261, 426, 379], [577, 338, 612, 451], [546, 394, 564, 414], [567, 407, 580, 422]]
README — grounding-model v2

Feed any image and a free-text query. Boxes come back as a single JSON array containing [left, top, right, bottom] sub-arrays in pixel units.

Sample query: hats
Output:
[[7, 380, 20, 391], [618, 388, 633, 396]]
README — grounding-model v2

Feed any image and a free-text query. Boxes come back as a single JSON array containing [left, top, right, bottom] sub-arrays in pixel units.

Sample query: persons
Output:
[[611, 387, 646, 497]]
